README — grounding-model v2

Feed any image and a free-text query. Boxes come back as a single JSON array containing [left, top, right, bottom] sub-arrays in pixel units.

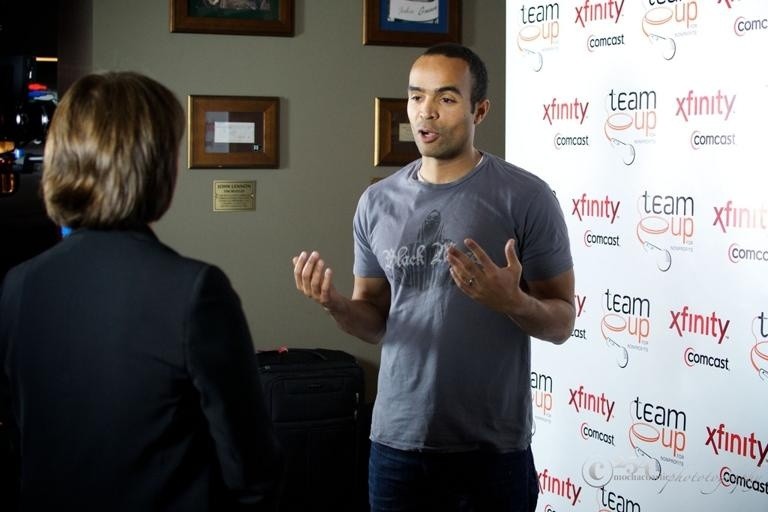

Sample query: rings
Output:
[[467, 273, 478, 287]]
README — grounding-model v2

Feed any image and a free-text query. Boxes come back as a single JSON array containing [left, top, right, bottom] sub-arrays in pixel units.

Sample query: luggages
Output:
[[254, 348, 367, 457]]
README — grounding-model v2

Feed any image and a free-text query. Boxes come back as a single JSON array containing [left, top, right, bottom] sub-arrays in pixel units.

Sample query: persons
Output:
[[1, 68, 279, 511], [291, 43, 576, 511]]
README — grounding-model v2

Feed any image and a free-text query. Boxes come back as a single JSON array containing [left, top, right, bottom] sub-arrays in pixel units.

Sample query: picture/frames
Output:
[[188, 94, 281, 172], [168, 0, 296, 40], [361, 0, 459, 47], [373, 96, 423, 168]]
